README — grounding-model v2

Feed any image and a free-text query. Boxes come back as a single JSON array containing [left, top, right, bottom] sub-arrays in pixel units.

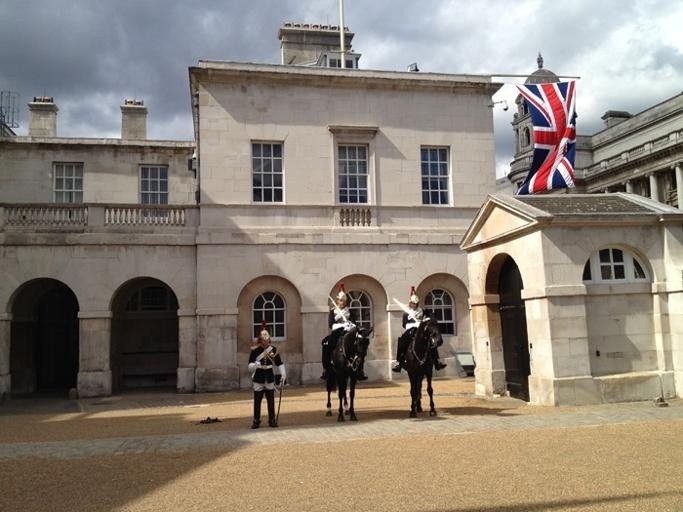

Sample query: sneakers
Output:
[[391, 362, 447, 372], [251, 418, 278, 429]]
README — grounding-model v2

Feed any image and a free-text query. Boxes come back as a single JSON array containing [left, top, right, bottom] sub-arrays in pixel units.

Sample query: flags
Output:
[[515, 81, 579, 196]]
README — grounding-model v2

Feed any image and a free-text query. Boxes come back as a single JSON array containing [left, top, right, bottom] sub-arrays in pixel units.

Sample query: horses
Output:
[[399, 307, 444, 420], [324, 323, 374, 423]]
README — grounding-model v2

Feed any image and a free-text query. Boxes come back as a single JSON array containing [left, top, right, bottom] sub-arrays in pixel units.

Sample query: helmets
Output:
[[337, 291, 347, 300], [260, 330, 269, 339], [409, 294, 420, 303]]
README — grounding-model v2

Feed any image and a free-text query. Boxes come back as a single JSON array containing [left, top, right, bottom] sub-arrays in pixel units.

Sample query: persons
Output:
[[248, 330, 287, 429], [392, 294, 447, 373], [320, 291, 369, 381]]
